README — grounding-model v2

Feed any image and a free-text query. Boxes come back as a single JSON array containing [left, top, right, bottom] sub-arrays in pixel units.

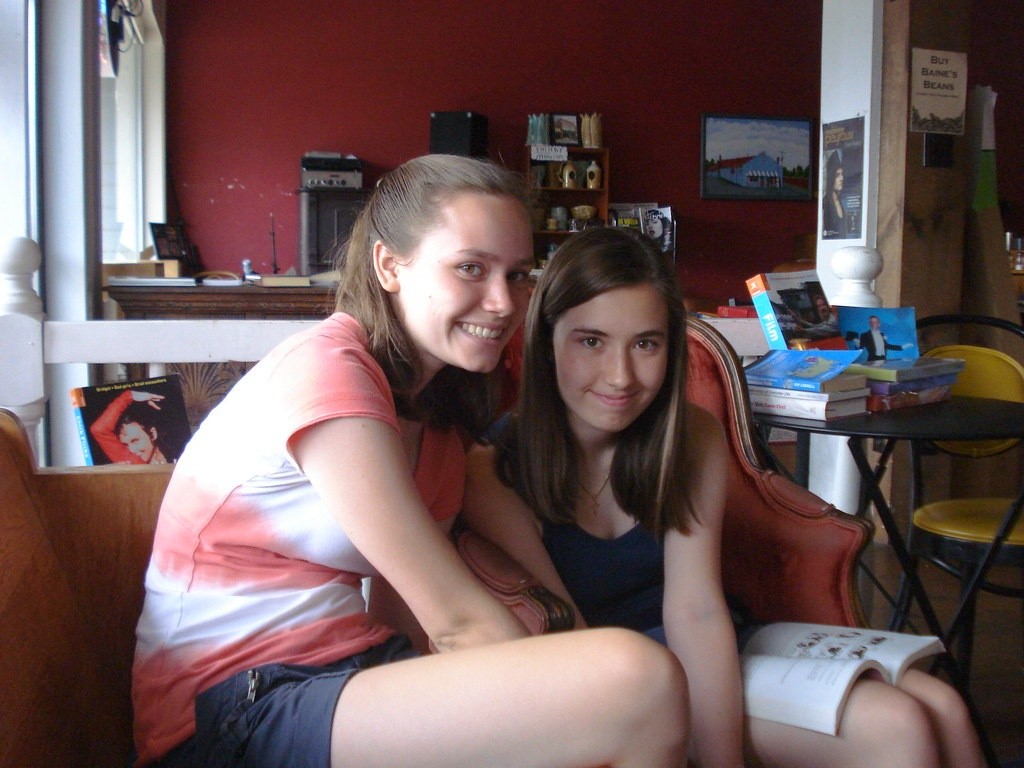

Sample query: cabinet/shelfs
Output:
[[522, 144, 608, 268]]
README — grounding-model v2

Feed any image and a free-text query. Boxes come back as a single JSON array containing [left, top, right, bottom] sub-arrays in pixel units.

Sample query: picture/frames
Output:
[[700, 112, 814, 203], [549, 112, 581, 146]]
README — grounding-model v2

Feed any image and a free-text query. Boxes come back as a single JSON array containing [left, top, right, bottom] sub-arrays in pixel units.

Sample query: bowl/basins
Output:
[[572, 206, 596, 219]]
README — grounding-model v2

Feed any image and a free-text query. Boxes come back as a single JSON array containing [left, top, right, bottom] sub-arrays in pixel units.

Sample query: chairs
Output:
[[514, 316, 870, 636], [0, 407, 176, 768], [887, 313, 1024, 693]]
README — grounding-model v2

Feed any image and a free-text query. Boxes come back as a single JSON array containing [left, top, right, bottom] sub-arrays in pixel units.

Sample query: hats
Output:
[[845, 331, 859, 341]]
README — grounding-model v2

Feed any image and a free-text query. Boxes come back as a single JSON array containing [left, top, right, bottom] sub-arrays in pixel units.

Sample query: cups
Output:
[[551, 207, 568, 230], [539, 260, 549, 269], [544, 219, 560, 230]]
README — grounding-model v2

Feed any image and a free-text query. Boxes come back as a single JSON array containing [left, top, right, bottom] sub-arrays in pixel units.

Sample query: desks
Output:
[[103, 282, 341, 435], [750, 394, 1024, 768]]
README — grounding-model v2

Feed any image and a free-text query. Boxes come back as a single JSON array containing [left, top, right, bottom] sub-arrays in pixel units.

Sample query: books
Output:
[[746, 270, 843, 351], [105, 275, 314, 288], [608, 202, 675, 259], [69, 373, 192, 467], [689, 306, 966, 422], [739, 620, 946, 736]]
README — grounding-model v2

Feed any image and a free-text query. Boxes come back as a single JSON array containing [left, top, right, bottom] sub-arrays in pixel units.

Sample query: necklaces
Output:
[[571, 469, 611, 517]]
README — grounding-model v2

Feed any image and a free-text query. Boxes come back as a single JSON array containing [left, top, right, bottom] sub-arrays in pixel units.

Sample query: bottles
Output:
[[562, 161, 576, 188], [586, 161, 601, 189]]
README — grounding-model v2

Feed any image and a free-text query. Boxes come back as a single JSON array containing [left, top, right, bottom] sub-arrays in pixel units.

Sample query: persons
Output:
[[92, 390, 167, 467], [489, 225, 987, 768], [131, 154, 688, 768], [785, 297, 840, 340], [823, 150, 848, 240], [644, 210, 674, 258], [852, 315, 914, 362]]
